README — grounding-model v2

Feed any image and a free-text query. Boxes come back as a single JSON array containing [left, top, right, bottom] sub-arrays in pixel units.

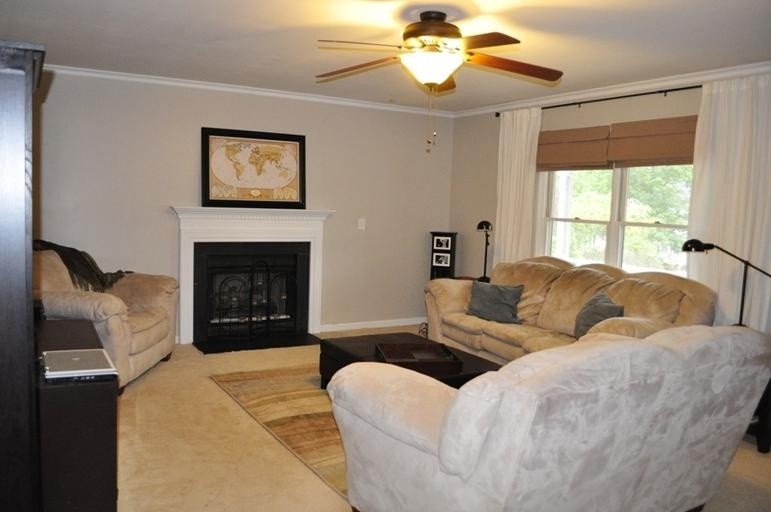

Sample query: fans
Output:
[[313, 33, 562, 98]]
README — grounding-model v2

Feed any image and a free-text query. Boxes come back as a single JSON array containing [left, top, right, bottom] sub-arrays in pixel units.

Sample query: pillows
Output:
[[574, 289, 624, 340], [466, 279, 524, 324]]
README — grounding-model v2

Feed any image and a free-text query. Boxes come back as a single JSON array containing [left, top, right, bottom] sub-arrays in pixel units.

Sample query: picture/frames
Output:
[[201, 125, 307, 208], [432, 253, 450, 267], [433, 236, 451, 250]]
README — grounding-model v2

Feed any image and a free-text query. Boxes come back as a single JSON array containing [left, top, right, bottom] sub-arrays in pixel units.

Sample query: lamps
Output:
[[682, 238, 767, 480], [477, 220, 493, 283], [401, 20, 465, 87]]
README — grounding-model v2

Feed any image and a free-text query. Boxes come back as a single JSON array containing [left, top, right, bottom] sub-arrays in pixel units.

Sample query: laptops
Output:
[[36, 348, 118, 383]]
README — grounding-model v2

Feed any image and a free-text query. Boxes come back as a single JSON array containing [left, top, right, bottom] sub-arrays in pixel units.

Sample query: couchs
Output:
[[424, 255, 719, 365], [326, 321, 771, 512], [33, 247, 178, 389]]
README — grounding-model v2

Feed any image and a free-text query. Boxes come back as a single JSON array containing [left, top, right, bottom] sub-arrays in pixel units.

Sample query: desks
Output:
[[32, 318, 119, 511]]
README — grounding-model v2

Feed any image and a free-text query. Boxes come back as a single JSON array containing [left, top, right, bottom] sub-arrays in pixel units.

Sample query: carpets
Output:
[[208, 360, 348, 503]]
